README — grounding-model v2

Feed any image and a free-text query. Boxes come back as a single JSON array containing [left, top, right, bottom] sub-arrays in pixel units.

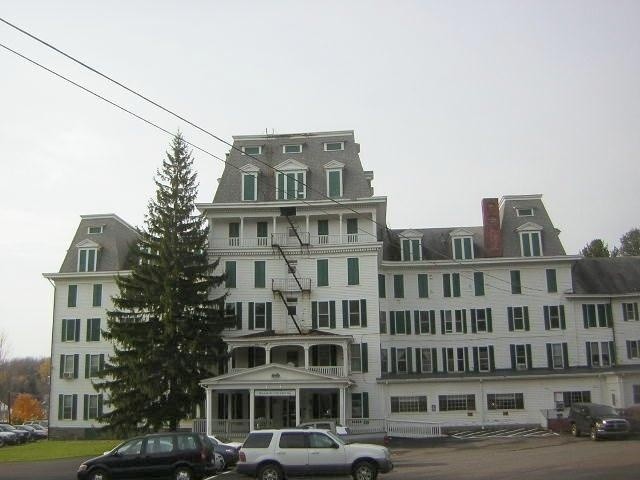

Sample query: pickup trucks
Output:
[[296, 420, 389, 446]]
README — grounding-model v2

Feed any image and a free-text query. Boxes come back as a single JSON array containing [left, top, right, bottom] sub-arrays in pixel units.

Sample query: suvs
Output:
[[568, 403, 630, 440], [236, 429, 393, 480]]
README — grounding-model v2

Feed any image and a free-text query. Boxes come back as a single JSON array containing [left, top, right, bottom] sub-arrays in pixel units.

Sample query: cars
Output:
[[0, 420, 48, 447], [77, 431, 242, 479]]
